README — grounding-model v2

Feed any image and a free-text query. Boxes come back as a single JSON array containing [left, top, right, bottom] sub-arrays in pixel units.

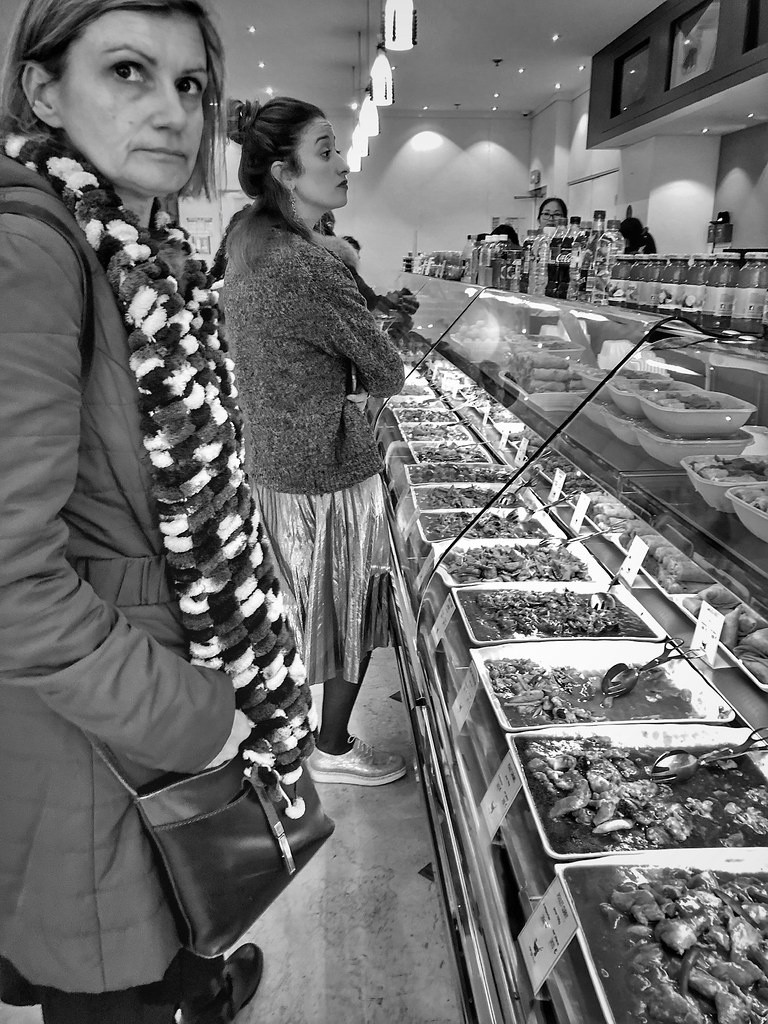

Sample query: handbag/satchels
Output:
[[76, 726, 336, 960]]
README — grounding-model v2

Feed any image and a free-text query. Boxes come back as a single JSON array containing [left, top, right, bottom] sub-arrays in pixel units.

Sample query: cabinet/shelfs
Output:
[[585, 0, 768, 153], [355, 267, 768, 1024]]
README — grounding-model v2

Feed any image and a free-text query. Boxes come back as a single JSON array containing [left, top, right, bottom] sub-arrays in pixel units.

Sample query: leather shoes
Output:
[[180, 942, 265, 1024]]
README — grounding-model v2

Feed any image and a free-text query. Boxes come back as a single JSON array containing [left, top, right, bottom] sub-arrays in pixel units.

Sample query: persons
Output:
[[218, 92, 409, 788], [538, 201, 572, 230], [339, 233, 377, 311], [4, 0, 317, 1022], [621, 215, 657, 257]]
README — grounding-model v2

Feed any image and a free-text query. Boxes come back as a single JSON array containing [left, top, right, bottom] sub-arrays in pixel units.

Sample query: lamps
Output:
[[369, 33, 396, 107], [379, 0, 419, 53], [345, 66, 364, 174], [350, 31, 369, 158], [358, 1, 380, 139]]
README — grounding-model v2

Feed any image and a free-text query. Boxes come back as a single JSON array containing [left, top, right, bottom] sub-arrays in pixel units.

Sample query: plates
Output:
[[384, 367, 768, 1024]]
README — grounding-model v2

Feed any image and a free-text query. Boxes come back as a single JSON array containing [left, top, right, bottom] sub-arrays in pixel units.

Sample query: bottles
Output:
[[463, 208, 768, 344]]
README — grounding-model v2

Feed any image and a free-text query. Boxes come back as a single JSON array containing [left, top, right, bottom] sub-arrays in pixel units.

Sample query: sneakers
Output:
[[306, 736, 407, 787]]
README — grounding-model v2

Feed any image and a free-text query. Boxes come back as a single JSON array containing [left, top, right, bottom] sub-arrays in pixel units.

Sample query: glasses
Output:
[[540, 213, 566, 221]]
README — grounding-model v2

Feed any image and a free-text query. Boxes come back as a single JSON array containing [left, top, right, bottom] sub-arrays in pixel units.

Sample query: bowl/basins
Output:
[[448, 320, 767, 545]]
